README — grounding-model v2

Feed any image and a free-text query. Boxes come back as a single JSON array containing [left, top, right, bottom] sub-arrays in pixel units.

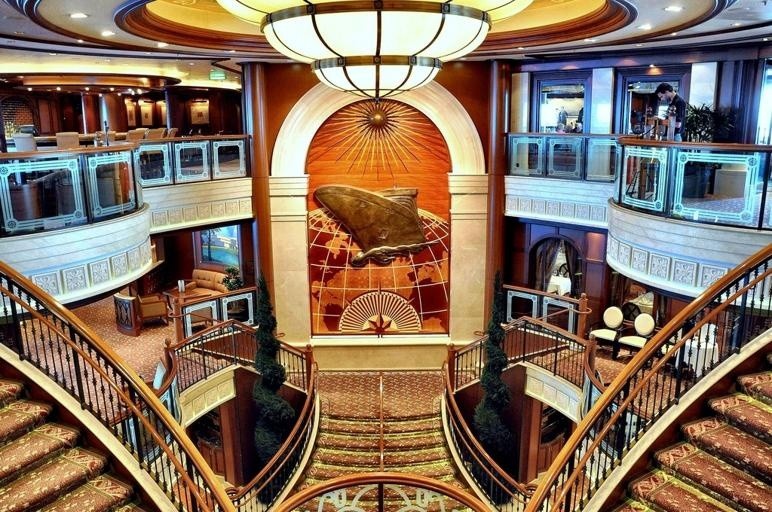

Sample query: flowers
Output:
[[222, 268, 243, 294]]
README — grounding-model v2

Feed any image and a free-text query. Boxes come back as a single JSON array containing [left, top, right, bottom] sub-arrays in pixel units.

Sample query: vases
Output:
[[230, 297, 238, 309]]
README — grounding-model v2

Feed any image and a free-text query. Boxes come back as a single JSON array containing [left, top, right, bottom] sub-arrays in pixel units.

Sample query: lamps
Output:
[[215, 0, 532, 106]]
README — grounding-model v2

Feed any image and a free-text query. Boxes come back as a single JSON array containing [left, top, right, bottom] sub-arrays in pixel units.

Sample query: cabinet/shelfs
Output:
[[195, 405, 225, 475], [538, 402, 566, 472]]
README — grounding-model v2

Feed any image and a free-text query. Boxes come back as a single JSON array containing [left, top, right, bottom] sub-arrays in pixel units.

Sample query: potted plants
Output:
[[469, 271, 513, 504], [250, 271, 296, 501]]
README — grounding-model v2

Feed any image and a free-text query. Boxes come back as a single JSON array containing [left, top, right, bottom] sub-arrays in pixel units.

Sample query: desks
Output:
[[673, 338, 720, 376], [163, 288, 213, 326]]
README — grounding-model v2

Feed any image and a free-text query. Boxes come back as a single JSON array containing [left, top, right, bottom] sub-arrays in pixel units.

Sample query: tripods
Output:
[[626, 158, 654, 200]]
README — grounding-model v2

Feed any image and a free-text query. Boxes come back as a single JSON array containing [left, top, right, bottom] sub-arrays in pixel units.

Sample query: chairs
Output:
[[129, 284, 168, 332], [13, 124, 179, 152], [586, 304, 657, 370]]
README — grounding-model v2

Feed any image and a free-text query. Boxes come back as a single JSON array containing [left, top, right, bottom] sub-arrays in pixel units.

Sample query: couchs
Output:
[[187, 268, 231, 303]]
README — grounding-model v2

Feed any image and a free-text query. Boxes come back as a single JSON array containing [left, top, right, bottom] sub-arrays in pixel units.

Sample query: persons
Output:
[[556, 106, 583, 133], [652, 83, 686, 143]]
